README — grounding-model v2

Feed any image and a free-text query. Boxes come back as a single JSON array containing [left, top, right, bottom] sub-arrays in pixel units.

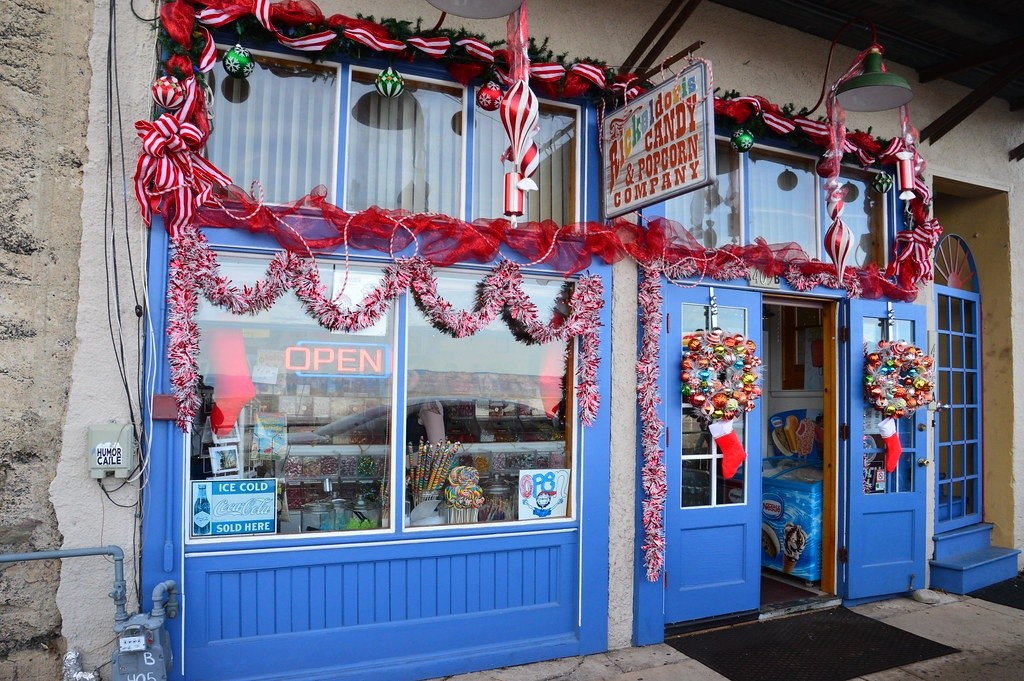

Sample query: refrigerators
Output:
[[723, 454, 824, 587]]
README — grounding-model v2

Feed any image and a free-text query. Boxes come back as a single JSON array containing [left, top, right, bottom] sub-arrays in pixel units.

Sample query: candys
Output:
[[445, 467, 485, 510]]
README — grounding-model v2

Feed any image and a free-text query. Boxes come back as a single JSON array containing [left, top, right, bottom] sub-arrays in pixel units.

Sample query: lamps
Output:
[[426, 0, 522, 19], [835, 42, 913, 112]]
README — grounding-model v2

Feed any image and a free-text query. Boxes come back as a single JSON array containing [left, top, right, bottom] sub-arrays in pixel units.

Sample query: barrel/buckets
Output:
[[300, 498, 378, 533], [482, 472, 513, 498]]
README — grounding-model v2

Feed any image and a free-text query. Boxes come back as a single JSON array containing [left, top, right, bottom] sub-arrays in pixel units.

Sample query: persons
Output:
[[406, 366, 445, 512], [220, 451, 226, 469]]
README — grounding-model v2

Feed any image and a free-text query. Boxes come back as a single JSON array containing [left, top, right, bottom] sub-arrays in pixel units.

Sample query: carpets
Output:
[[661, 605, 963, 680], [965, 574, 1024, 612]]
